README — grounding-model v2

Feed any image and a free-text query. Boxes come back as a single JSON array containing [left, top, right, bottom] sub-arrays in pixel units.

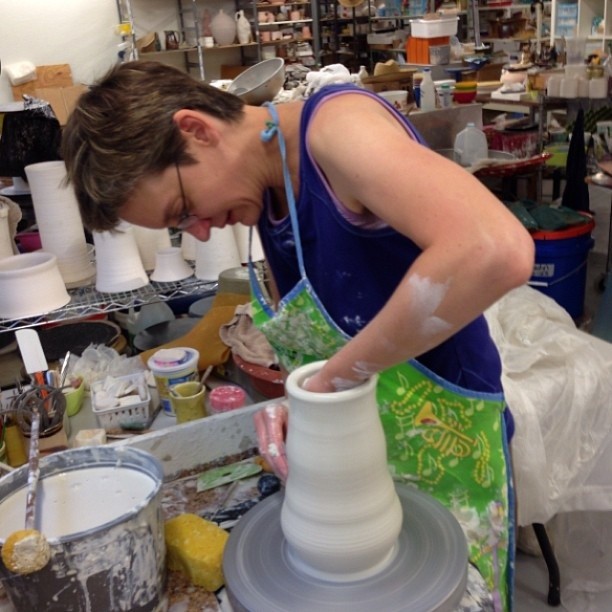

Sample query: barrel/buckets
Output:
[[519, 211, 594, 326]]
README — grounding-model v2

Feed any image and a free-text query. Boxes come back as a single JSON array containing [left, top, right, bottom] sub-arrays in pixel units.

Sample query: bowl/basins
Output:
[[453, 80, 478, 104], [477, 81, 505, 95], [226, 56, 285, 107]]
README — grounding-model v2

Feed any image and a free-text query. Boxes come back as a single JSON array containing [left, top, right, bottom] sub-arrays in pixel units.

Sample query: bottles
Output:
[[420, 68, 436, 112], [440, 83, 452, 109]]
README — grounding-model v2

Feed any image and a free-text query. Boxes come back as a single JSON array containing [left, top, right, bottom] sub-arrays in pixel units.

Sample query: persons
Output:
[[61, 61, 535, 609]]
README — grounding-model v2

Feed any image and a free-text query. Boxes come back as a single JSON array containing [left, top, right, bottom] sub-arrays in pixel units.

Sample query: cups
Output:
[[166, 381, 207, 426]]
